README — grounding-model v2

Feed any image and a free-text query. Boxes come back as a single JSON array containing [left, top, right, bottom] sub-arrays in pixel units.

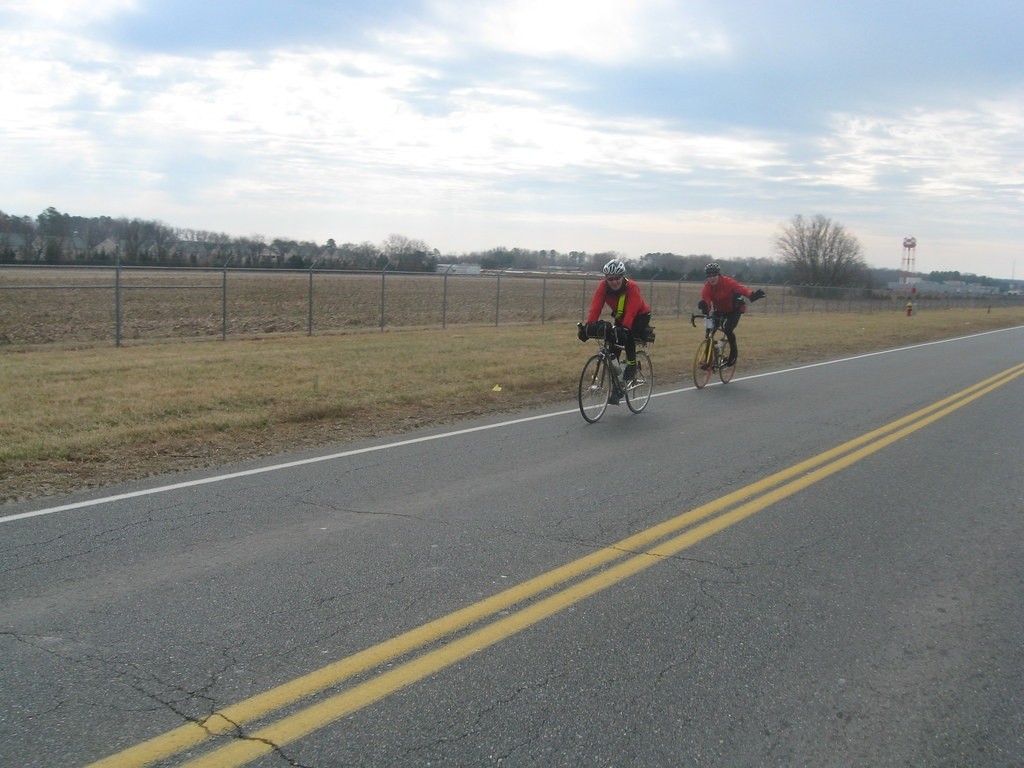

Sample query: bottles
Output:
[[617, 359, 626, 382], [714, 340, 719, 357], [610, 352, 619, 368], [718, 339, 723, 356]]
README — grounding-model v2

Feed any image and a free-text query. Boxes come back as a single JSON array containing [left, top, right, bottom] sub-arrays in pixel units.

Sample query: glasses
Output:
[[707, 273, 717, 277], [606, 275, 621, 281]]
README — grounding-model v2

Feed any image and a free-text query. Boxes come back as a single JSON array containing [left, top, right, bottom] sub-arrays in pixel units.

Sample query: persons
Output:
[[578, 259, 651, 380], [698, 263, 767, 369]]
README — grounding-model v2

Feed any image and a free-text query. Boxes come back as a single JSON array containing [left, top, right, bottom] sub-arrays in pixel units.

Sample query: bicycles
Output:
[[691, 313, 737, 389], [577, 322, 654, 424]]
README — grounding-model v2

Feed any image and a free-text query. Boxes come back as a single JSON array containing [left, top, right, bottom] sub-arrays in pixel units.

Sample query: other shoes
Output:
[[623, 364, 637, 379], [727, 351, 738, 366], [608, 388, 623, 405]]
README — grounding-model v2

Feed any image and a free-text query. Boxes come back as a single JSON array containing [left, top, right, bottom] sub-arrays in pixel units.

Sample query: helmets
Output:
[[603, 259, 625, 274], [704, 263, 720, 273]]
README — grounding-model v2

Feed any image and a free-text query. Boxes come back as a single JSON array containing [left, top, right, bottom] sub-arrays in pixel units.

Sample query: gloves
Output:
[[619, 327, 630, 346], [577, 323, 589, 342], [752, 289, 767, 301], [698, 301, 707, 309]]
